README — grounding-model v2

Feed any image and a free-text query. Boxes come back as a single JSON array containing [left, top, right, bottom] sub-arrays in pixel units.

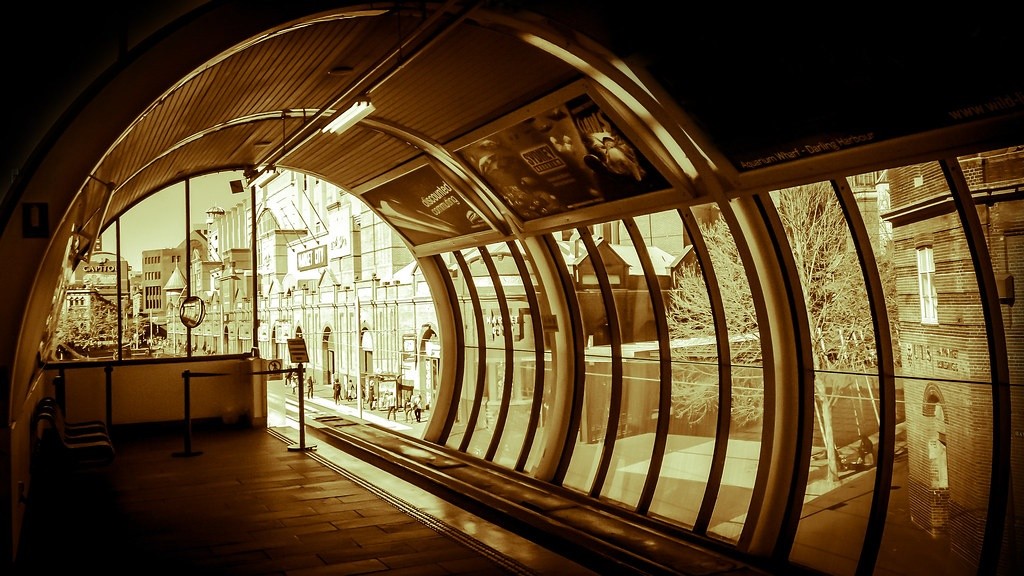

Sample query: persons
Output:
[[333, 379, 343, 401], [858, 434, 873, 464], [414, 393, 423, 423], [362, 386, 366, 409], [369, 387, 375, 410], [386, 392, 397, 422], [152, 335, 216, 356], [284, 365, 293, 387], [404, 397, 414, 424], [307, 376, 314, 399], [347, 379, 355, 402], [291, 376, 298, 395]]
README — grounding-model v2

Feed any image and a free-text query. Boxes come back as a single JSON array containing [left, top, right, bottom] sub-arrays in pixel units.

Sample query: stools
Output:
[[808, 467, 821, 483], [810, 458, 828, 478]]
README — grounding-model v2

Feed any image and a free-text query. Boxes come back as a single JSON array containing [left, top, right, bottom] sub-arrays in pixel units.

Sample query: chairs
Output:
[[35, 403, 112, 443], [38, 394, 109, 435], [32, 413, 117, 470]]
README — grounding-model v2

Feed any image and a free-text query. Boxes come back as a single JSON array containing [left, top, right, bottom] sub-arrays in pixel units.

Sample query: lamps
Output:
[[247, 163, 277, 188], [319, 90, 376, 135]]
